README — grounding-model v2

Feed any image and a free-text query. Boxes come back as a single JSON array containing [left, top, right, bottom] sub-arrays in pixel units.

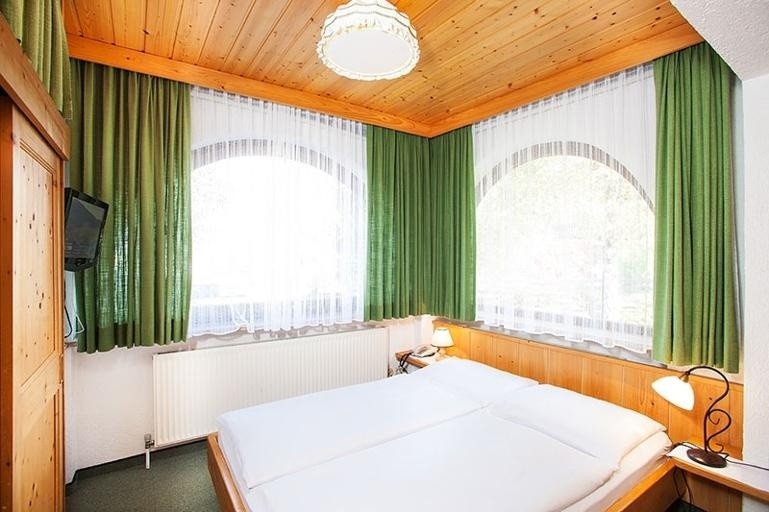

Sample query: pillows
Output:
[[489, 384, 666, 473], [421, 355, 538, 408]]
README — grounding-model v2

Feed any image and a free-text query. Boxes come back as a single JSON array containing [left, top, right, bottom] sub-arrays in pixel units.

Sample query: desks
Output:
[[670, 442, 769, 504], [396, 349, 430, 373]]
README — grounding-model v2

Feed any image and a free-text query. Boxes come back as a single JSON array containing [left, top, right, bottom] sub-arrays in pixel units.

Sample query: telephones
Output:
[[412, 344, 438, 357]]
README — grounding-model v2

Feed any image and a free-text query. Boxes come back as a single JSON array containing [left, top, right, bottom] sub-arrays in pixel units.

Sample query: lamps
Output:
[[316, 0, 421, 81], [431, 328, 453, 362], [651, 365, 731, 468]]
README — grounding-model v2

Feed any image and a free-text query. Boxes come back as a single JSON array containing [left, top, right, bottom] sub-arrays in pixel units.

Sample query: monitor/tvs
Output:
[[65, 187, 109, 272]]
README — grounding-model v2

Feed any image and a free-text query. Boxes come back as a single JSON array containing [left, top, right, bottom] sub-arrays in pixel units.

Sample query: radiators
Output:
[[144, 327, 391, 469]]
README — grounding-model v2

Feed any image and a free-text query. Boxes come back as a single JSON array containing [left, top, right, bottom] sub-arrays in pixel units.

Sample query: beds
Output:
[[207, 317, 743, 512]]
